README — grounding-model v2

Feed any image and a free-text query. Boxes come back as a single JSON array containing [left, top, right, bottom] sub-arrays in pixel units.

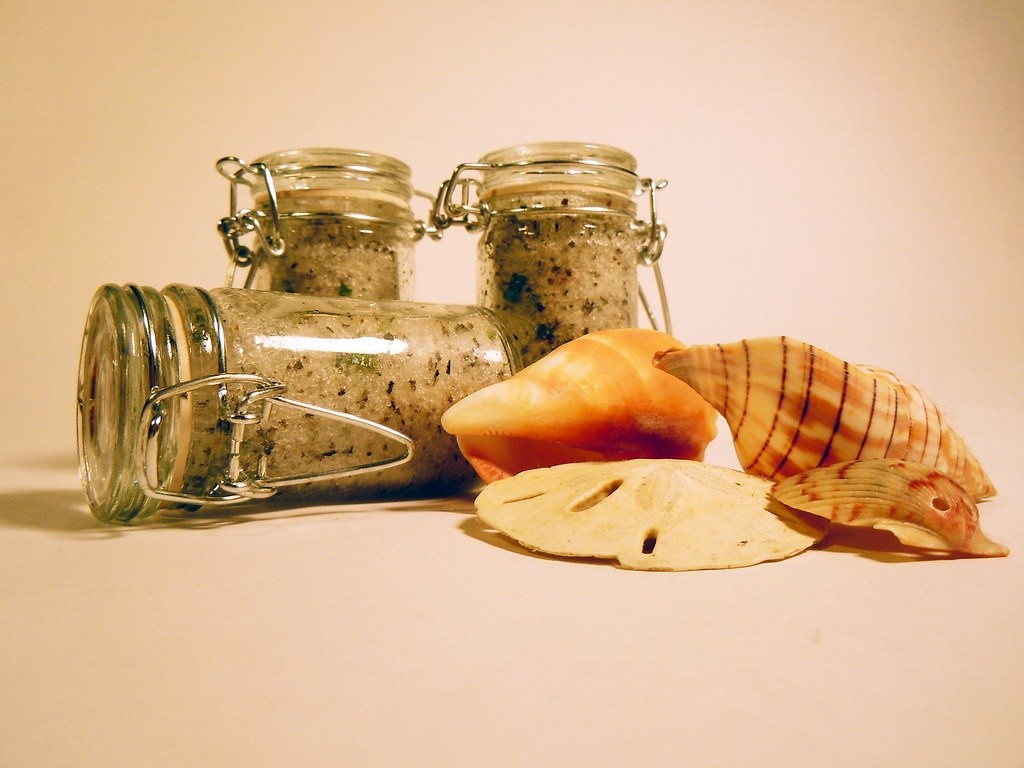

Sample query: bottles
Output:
[[215, 147, 443, 300], [444, 139, 669, 368], [75, 286, 524, 526]]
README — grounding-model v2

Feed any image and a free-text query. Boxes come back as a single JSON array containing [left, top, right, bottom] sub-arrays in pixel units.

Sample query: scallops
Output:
[[768, 455, 1012, 560]]
